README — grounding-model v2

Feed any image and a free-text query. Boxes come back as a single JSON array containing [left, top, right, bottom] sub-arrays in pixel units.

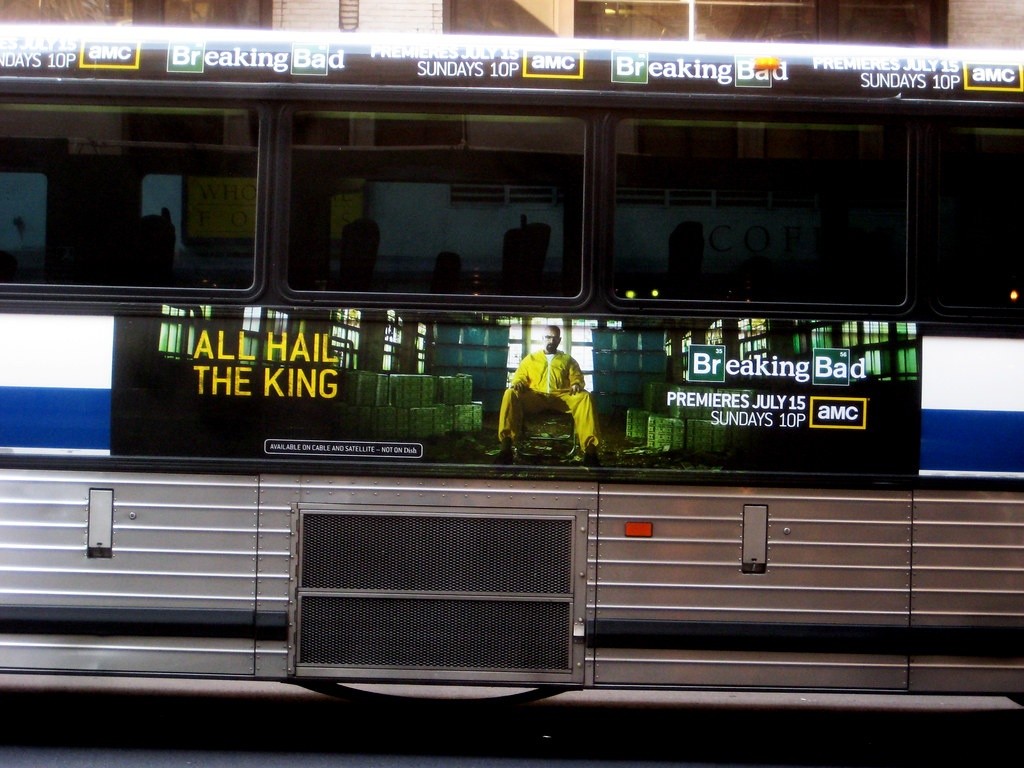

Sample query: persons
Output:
[[492, 325, 604, 467]]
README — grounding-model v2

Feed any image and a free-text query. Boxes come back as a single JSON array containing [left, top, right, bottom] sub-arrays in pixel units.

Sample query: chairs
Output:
[[46, 195, 820, 300], [518, 411, 576, 460]]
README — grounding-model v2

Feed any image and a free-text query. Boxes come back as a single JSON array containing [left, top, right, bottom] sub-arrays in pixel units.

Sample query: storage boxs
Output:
[[626, 383, 758, 453], [343, 369, 481, 437]]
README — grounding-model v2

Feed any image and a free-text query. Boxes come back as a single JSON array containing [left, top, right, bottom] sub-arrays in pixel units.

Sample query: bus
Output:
[[0, 18, 1024, 715]]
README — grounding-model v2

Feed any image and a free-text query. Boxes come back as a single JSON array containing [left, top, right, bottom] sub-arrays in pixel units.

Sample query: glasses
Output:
[[545, 335, 559, 341]]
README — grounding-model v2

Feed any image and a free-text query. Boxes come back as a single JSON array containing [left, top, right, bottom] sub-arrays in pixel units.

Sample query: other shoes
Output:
[[496, 437, 514, 465], [584, 445, 601, 467]]
